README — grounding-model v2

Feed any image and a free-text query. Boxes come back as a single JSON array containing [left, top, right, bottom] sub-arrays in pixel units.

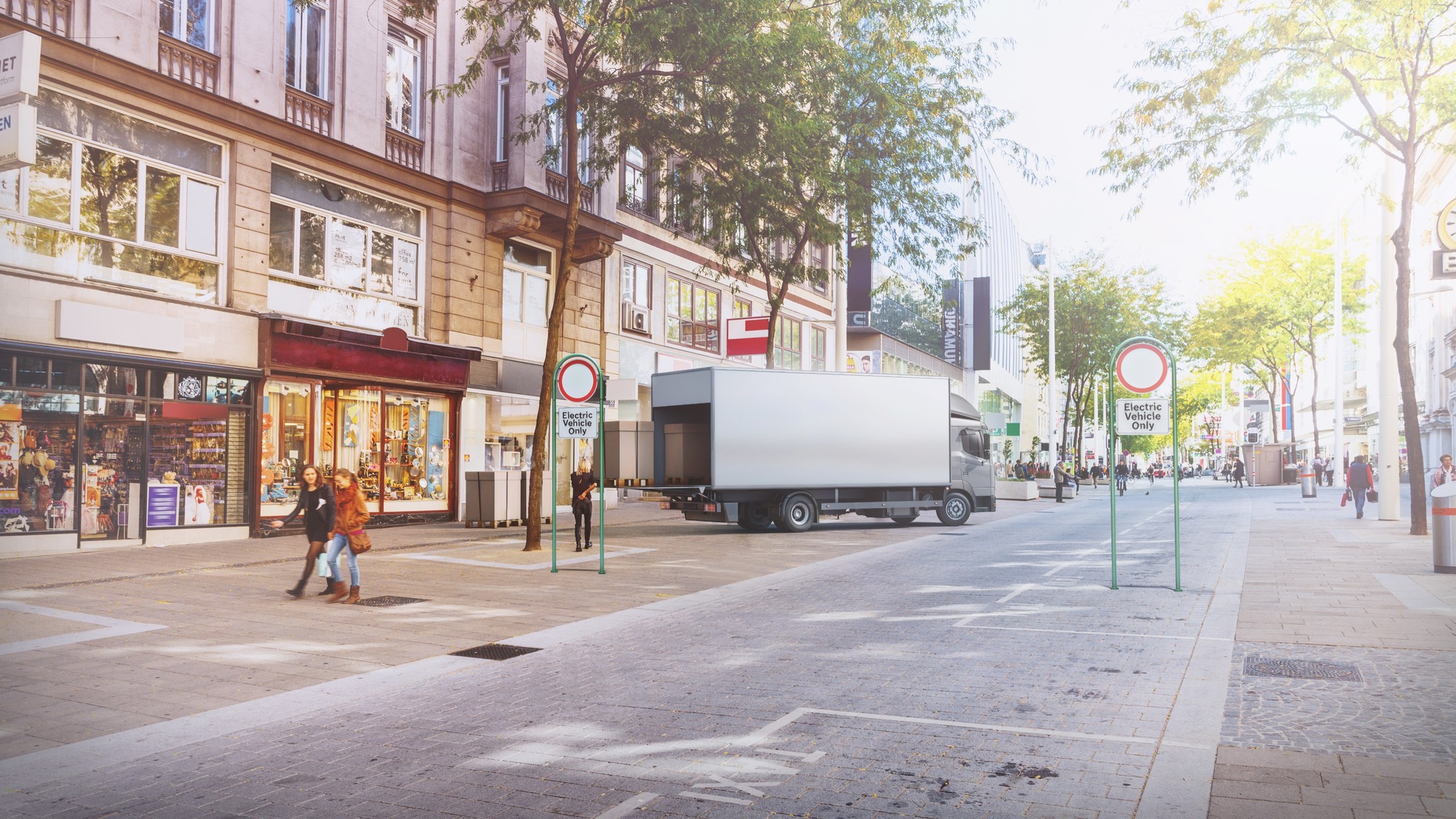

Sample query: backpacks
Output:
[[1017, 465, 1024, 478]]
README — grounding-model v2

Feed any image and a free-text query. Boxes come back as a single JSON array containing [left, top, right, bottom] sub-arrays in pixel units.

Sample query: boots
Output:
[[584, 525, 592, 549], [342, 585, 361, 604], [575, 525, 583, 552], [325, 580, 350, 603], [318, 577, 336, 595], [286, 579, 306, 598]]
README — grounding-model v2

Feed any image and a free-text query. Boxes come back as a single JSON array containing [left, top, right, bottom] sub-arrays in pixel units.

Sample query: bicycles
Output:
[[1116, 472, 1135, 496]]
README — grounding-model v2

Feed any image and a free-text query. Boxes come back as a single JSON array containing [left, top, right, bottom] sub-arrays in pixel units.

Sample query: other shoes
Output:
[[1320, 485, 1323, 486], [1327, 485, 1332, 486], [1117, 486, 1119, 490], [1233, 486, 1236, 488], [1230, 480, 1232, 482], [1357, 512, 1364, 519], [1227, 480, 1229, 482], [1124, 486, 1127, 490], [1239, 486, 1243, 488]]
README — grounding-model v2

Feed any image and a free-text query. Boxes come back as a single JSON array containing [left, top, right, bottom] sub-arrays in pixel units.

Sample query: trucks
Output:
[[651, 367, 997, 527]]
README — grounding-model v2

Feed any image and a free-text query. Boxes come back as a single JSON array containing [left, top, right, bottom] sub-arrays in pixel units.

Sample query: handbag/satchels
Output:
[[1314, 463, 1321, 470], [318, 539, 341, 577], [1341, 487, 1352, 507], [1366, 490, 1378, 503], [348, 531, 371, 554]]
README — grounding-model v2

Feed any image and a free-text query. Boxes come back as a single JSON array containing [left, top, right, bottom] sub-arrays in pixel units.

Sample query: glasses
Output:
[[334, 478, 345, 484], [1443, 459, 1452, 462]]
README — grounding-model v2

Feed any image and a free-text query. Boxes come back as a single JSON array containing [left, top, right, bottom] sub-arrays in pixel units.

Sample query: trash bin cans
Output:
[[1301, 469, 1317, 498], [1430, 480, 1456, 575]]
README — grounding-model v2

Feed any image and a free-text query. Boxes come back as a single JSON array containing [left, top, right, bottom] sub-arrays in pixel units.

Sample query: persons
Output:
[[1399, 456, 1402, 482], [1090, 462, 1100, 488], [270, 464, 337, 597], [1297, 453, 1348, 487], [325, 468, 370, 604], [570, 459, 597, 551], [0, 422, 17, 488], [1350, 456, 1356, 466], [1062, 456, 1238, 485], [1115, 460, 1129, 491], [994, 462, 997, 476], [1066, 468, 1079, 494], [1431, 454, 1456, 488], [1346, 455, 1374, 519], [192, 488, 210, 525], [1062, 466, 1077, 496], [1007, 460, 1015, 478], [1367, 458, 1374, 474], [1014, 459, 1049, 481], [1053, 461, 1065, 503], [1283, 454, 1289, 469], [1233, 459, 1244, 488]]
